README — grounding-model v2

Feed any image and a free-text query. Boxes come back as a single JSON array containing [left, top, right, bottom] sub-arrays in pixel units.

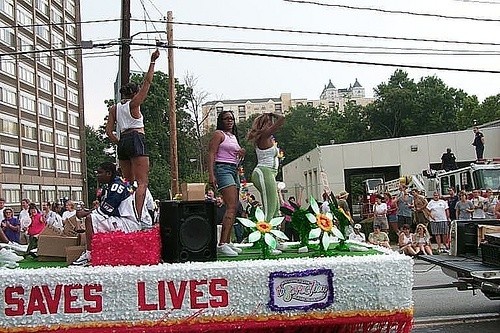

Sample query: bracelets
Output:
[[151, 62, 155, 64]]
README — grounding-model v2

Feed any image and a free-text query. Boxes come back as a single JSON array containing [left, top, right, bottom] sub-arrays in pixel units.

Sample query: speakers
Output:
[[160, 201, 217, 263]]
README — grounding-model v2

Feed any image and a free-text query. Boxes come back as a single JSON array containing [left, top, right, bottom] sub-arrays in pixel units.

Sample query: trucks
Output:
[[356, 157, 500, 225]]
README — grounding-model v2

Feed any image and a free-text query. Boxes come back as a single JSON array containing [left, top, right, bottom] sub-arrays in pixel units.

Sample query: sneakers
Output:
[[72, 250, 88, 264], [83, 251, 92, 267]]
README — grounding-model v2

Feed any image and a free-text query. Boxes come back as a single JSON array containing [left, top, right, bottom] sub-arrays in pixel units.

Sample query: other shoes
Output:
[[437, 248, 450, 253], [270, 249, 282, 256], [217, 243, 238, 256], [225, 242, 242, 254]]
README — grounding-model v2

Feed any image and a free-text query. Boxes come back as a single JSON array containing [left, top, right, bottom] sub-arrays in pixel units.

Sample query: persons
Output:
[[424, 192, 451, 253], [411, 189, 430, 227], [368, 224, 390, 248], [372, 193, 398, 233], [106, 48, 160, 230], [396, 184, 415, 228], [0, 197, 99, 245], [207, 188, 259, 243], [471, 127, 486, 159], [349, 223, 365, 242], [133, 179, 154, 224], [319, 190, 354, 223], [246, 112, 285, 254], [68, 161, 141, 269], [278, 185, 304, 242], [398, 223, 434, 256], [209, 110, 246, 256], [441, 148, 459, 172], [446, 187, 500, 221]]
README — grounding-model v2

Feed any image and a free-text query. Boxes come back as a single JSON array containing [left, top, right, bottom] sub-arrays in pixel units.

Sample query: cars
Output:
[[352, 204, 361, 214]]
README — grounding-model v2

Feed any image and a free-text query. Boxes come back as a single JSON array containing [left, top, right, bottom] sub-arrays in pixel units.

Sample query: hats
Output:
[[354, 224, 361, 229], [339, 191, 349, 198]]
